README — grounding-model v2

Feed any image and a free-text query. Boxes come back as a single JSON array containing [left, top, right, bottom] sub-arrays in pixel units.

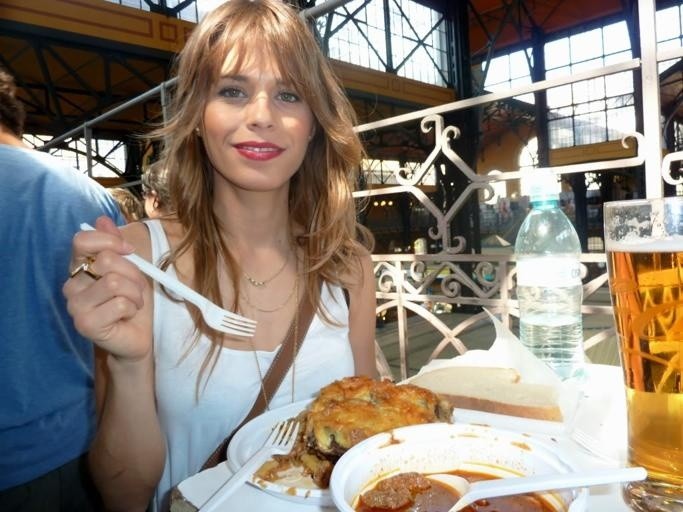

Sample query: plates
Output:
[[228, 397, 337, 507]]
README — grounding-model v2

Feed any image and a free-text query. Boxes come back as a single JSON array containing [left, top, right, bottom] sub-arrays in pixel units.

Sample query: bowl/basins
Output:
[[329, 422, 590, 512]]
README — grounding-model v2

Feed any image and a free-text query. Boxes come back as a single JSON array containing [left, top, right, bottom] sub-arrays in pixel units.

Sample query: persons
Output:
[[138, 157, 182, 223], [99, 185, 145, 229], [0, 63, 135, 512], [56, 1, 385, 512]]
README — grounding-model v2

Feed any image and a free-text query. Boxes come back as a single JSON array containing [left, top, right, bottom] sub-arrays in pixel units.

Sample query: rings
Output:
[[68, 253, 103, 281]]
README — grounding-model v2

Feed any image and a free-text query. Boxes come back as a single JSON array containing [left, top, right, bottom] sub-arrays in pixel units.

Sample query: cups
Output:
[[602, 197, 683, 511]]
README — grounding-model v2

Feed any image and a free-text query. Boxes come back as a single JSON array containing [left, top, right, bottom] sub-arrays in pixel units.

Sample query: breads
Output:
[[288, 375, 455, 488]]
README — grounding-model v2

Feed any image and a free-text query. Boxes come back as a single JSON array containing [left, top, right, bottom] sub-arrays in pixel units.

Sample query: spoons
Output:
[[423, 466, 647, 511]]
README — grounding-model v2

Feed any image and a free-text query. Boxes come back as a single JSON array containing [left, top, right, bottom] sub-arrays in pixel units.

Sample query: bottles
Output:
[[515, 174, 584, 384]]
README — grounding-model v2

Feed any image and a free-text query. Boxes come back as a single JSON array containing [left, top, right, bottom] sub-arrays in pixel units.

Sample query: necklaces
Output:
[[213, 228, 304, 412]]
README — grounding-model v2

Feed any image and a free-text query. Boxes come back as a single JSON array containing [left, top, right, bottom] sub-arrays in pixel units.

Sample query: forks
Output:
[[194, 421, 300, 511], [79, 220, 258, 340]]
